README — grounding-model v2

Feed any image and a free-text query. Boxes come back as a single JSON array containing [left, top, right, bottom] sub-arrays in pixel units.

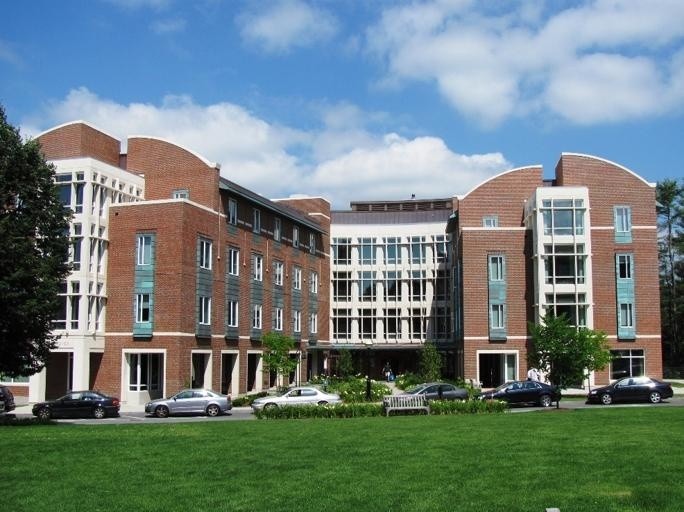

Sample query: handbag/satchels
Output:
[[389, 375, 394, 381]]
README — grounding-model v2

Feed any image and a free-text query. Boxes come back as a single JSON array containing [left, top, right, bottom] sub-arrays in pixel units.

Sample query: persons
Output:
[[381, 360, 392, 383]]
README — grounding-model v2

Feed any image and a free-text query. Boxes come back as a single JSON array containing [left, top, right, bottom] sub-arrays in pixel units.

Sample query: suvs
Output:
[[0, 384, 17, 413]]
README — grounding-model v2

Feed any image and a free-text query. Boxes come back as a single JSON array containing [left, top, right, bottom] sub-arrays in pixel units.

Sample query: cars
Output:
[[251, 386, 343, 415], [395, 376, 673, 407], [144, 389, 233, 419], [32, 391, 121, 421]]
